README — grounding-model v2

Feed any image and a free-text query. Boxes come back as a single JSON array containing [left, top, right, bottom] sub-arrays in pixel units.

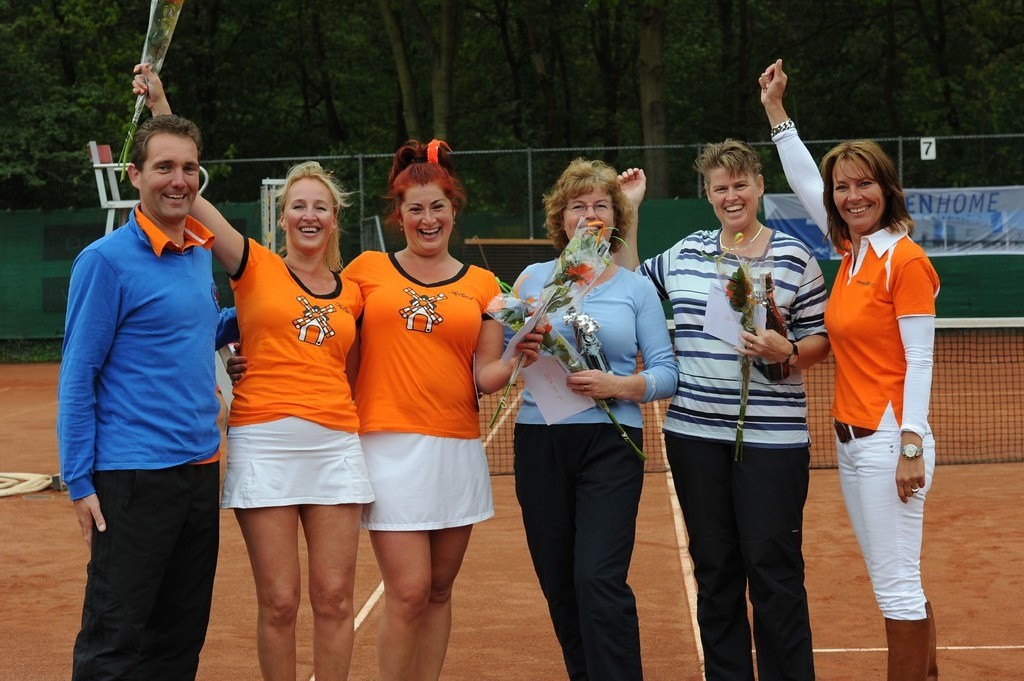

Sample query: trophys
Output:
[[561, 309, 618, 406]]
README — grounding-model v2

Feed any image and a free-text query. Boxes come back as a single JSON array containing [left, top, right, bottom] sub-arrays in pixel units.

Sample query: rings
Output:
[[749, 343, 753, 349], [535, 344, 540, 352], [751, 335, 757, 342], [583, 384, 589, 391], [911, 488, 919, 493]]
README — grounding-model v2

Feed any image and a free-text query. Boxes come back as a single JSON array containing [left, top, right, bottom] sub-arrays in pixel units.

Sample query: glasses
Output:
[[565, 202, 614, 214]]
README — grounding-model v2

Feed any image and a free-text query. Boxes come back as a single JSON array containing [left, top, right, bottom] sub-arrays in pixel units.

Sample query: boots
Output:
[[885, 615, 928, 681], [924, 601, 938, 681]]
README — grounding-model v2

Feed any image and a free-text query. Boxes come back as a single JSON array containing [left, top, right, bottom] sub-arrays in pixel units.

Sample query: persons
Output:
[[502, 151, 683, 681], [341, 135, 547, 679], [132, 49, 378, 681], [55, 112, 242, 681], [615, 135, 838, 679], [753, 56, 946, 680]]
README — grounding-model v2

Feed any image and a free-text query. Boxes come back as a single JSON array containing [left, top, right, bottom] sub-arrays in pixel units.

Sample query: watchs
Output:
[[783, 342, 800, 367], [899, 443, 924, 460]]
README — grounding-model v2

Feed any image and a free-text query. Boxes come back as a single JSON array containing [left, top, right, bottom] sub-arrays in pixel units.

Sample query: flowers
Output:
[[717, 232, 767, 460], [488, 216, 624, 428], [485, 291, 648, 464], [116, 0, 186, 183]]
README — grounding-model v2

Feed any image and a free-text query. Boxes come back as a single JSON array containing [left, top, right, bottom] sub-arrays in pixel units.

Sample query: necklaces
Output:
[[718, 224, 765, 253]]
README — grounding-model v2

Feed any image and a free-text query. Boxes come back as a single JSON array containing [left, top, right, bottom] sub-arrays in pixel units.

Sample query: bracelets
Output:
[[769, 116, 796, 138]]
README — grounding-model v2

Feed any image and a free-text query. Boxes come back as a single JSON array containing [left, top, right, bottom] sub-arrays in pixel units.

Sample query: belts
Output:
[[834, 419, 876, 445]]
